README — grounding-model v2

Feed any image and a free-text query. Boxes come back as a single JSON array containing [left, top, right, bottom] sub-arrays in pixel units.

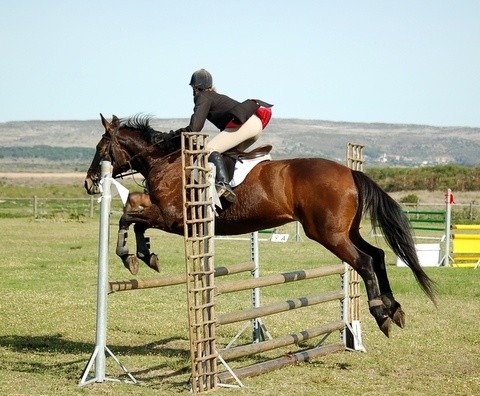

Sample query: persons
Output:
[[162, 68, 275, 204]]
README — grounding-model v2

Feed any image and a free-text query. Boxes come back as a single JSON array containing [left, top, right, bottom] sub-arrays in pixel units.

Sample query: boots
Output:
[[207, 151, 237, 203]]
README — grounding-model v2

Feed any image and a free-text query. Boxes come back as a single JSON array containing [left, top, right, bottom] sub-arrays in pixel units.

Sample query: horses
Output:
[[84, 113, 441, 339]]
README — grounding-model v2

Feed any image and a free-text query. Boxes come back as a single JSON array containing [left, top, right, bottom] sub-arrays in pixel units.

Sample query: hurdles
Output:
[[179, 130, 366, 392], [76, 160, 276, 384]]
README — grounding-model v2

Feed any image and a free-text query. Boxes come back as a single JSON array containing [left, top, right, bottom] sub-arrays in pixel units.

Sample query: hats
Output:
[[189, 69, 212, 90]]
